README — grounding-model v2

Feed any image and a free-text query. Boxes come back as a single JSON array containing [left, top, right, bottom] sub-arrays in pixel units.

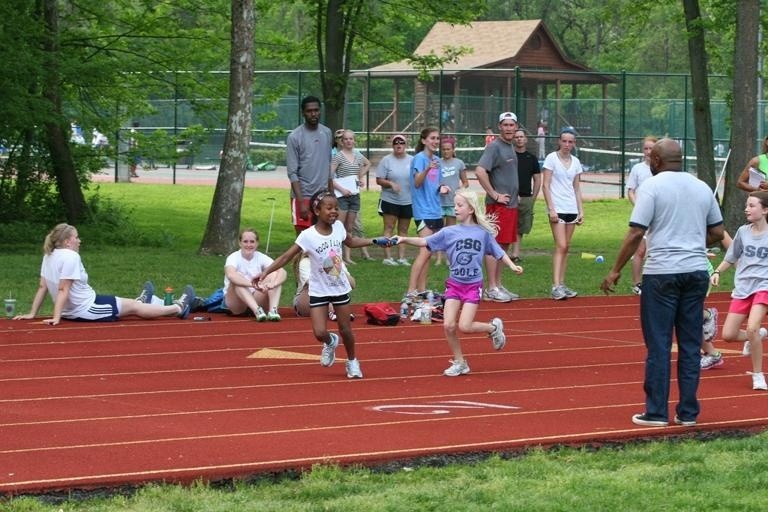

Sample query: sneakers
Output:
[[632, 284, 642, 295], [703, 307, 718, 342], [135, 281, 154, 304], [321, 332, 338, 367], [699, 351, 724, 369], [382, 258, 411, 265], [752, 371, 768, 390], [444, 358, 470, 376], [743, 327, 767, 355], [346, 357, 362, 378], [172, 285, 194, 319], [552, 284, 577, 300], [483, 287, 520, 302], [673, 414, 697, 427], [254, 306, 267, 322], [632, 413, 669, 427], [267, 312, 281, 320], [489, 319, 506, 350]]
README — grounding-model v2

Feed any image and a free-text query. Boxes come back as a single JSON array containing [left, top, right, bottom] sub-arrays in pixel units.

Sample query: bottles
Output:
[[596, 256, 603, 263], [427, 289, 434, 303], [163, 286, 175, 305], [400, 299, 409, 319], [420, 299, 432, 325], [4, 299, 17, 318]]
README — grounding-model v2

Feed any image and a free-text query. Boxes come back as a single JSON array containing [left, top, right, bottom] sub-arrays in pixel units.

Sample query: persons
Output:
[[251, 188, 387, 378], [541, 131, 583, 301], [10, 220, 196, 328], [474, 111, 545, 302], [376, 135, 414, 266], [434, 139, 469, 266], [129, 120, 144, 179], [91, 127, 110, 148], [600, 138, 725, 426], [222, 229, 287, 321], [286, 95, 334, 302], [329, 127, 376, 264], [626, 136, 768, 393], [292, 254, 356, 322], [389, 188, 523, 379], [409, 126, 446, 295]]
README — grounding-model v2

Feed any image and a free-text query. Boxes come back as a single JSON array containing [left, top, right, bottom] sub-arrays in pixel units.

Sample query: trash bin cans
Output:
[[176, 139, 194, 166]]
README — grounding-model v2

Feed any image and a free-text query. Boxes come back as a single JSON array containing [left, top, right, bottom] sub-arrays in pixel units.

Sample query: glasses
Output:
[[394, 140, 405, 145]]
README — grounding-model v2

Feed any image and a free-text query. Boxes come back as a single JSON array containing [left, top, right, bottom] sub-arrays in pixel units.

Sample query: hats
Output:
[[500, 111, 518, 124], [392, 135, 407, 144]]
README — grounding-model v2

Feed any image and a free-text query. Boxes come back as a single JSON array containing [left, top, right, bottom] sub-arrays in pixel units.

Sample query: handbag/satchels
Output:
[[367, 302, 400, 320]]
